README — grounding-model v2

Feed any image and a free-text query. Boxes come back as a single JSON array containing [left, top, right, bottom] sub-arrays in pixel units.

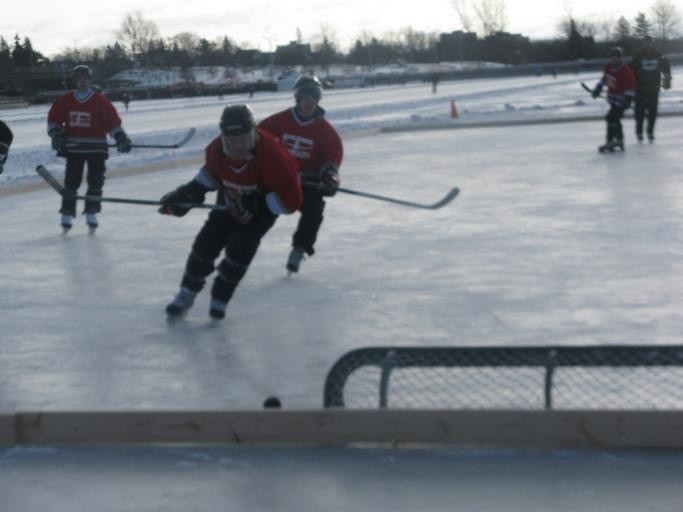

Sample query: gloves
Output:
[[159, 179, 208, 216], [315, 169, 338, 195], [49, 128, 66, 153], [117, 132, 132, 152], [226, 193, 261, 225]]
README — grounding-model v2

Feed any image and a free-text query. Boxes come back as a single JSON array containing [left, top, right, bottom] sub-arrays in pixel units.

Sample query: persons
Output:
[[44, 63, 132, 231], [590, 45, 637, 149], [255, 75, 343, 272], [625, 33, 672, 142], [155, 102, 305, 320]]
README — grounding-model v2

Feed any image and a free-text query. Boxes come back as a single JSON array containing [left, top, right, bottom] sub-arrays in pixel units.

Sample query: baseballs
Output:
[[264, 396, 281, 408]]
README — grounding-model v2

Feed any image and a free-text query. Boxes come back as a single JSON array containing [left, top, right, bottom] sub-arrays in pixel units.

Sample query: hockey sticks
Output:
[[581, 81, 635, 110], [336, 184, 459, 209], [72, 127, 197, 149], [36, 164, 226, 213]]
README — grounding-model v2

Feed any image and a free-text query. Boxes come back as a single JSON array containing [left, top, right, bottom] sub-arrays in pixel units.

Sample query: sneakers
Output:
[[167, 291, 194, 314], [210, 296, 227, 318], [62, 212, 98, 227], [287, 248, 304, 272]]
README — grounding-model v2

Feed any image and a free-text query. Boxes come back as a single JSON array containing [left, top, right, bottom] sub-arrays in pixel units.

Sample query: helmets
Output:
[[293, 75, 322, 102], [71, 65, 93, 83], [220, 104, 253, 135]]
